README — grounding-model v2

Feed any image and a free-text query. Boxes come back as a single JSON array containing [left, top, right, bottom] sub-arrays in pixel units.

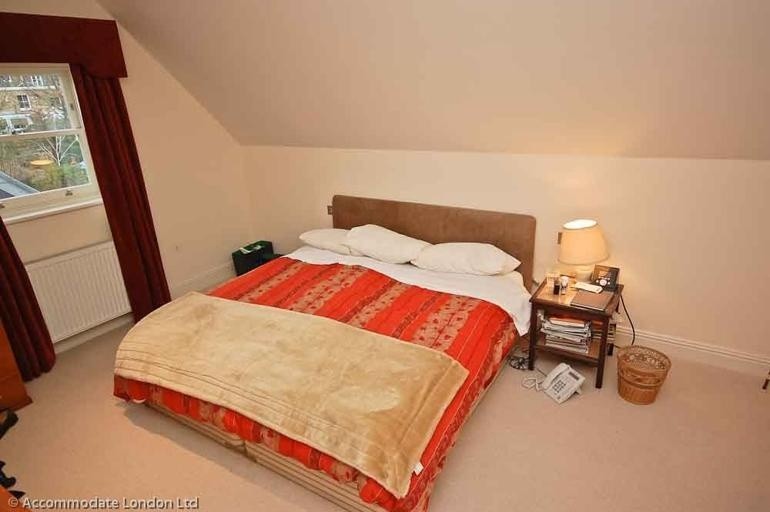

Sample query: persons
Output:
[[596, 276, 611, 286]]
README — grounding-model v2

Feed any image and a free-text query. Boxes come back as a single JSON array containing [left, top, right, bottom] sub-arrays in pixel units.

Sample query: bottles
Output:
[[554, 276, 568, 295]]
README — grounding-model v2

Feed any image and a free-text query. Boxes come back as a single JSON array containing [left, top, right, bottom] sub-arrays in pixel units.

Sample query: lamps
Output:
[[558, 219, 611, 291]]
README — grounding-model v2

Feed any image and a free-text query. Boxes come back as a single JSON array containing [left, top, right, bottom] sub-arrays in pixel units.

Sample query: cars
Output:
[[0, 113, 33, 135]]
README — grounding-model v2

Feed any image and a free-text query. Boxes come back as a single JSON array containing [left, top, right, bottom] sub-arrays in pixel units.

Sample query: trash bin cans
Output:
[[232, 241, 274, 274]]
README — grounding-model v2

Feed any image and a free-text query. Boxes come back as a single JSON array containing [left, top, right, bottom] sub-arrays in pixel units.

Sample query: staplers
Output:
[[560, 276, 569, 295]]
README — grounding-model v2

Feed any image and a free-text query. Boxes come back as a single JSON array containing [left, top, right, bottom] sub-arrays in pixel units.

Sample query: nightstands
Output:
[[528, 277, 625, 389]]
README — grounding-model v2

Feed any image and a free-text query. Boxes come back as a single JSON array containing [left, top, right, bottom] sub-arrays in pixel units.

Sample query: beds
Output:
[[115, 196, 536, 512]]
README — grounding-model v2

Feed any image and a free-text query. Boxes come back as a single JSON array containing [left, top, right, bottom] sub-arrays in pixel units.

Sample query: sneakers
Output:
[[1, 408, 18, 440]]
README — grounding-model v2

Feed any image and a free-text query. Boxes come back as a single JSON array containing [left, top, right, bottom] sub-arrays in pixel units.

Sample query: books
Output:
[[540, 311, 624, 353], [570, 289, 615, 311]]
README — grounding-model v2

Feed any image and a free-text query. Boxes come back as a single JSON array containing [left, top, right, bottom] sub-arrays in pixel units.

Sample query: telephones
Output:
[[541, 362, 586, 404]]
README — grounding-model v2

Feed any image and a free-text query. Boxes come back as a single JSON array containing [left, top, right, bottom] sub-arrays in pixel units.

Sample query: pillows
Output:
[[341, 224, 422, 262], [413, 242, 520, 276], [301, 227, 361, 255]]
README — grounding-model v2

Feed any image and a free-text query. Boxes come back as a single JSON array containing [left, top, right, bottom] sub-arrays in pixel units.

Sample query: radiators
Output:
[[25, 240, 132, 344]]
